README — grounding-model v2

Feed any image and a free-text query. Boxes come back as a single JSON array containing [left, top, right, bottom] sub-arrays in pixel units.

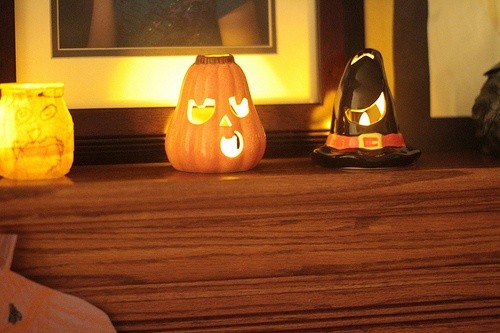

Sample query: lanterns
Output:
[[163, 53, 269, 174], [0, 81, 77, 181]]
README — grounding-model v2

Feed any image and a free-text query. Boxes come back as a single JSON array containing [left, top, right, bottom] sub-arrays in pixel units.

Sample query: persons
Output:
[[85, 1, 262, 48]]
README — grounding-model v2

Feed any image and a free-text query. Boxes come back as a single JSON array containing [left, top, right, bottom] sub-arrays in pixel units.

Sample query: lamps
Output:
[[0, 81, 75, 181], [166, 52, 267, 173], [310, 49, 422, 169]]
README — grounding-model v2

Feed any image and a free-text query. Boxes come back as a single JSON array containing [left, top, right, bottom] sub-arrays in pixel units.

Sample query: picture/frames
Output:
[[51, 0, 278, 57]]
